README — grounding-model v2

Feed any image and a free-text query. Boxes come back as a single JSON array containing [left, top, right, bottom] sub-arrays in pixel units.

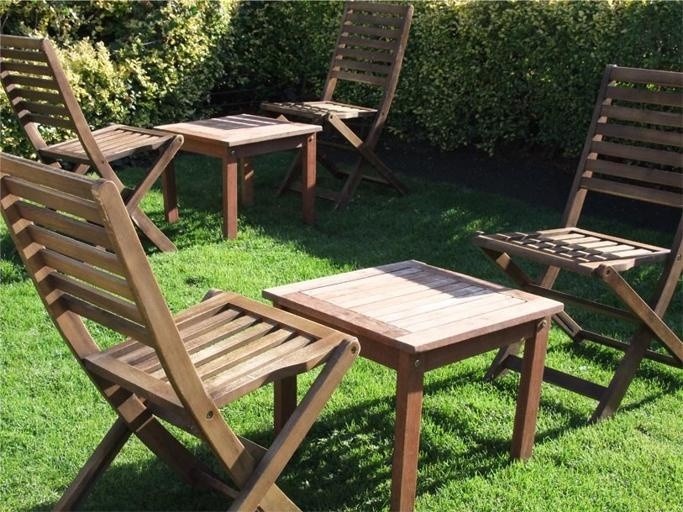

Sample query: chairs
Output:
[[474, 65, 683, 425], [0, 154, 359, 511], [255, 2, 413, 214], [1, 33, 186, 260]]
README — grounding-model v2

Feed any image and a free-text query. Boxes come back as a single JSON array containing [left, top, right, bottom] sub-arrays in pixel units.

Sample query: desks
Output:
[[151, 113, 325, 243], [259, 258, 566, 512]]
